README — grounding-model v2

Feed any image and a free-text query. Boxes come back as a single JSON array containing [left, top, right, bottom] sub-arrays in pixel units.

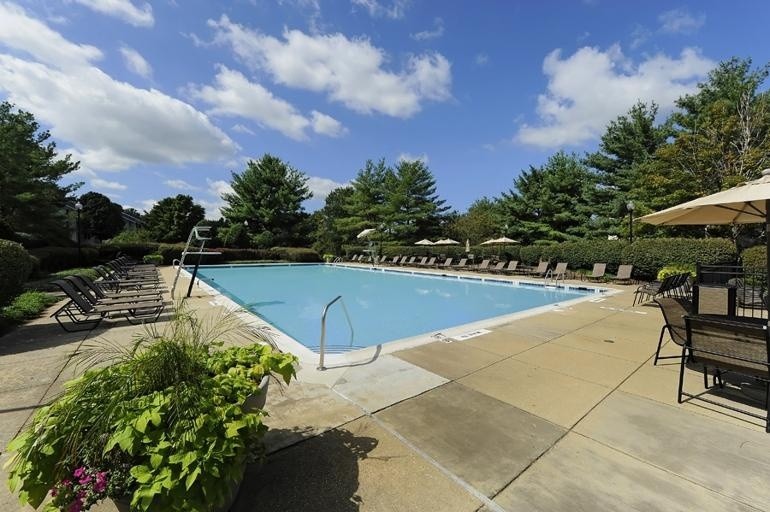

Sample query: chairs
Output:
[[532, 262, 548, 278], [452, 259, 468, 270], [505, 260, 518, 275], [49, 258, 163, 333], [654, 294, 770, 410], [545, 263, 568, 280], [611, 264, 633, 284], [493, 262, 506, 274], [726, 286, 769, 310], [351, 254, 436, 270], [585, 263, 607, 283], [476, 259, 490, 274], [439, 258, 453, 270], [631, 271, 692, 308]]
